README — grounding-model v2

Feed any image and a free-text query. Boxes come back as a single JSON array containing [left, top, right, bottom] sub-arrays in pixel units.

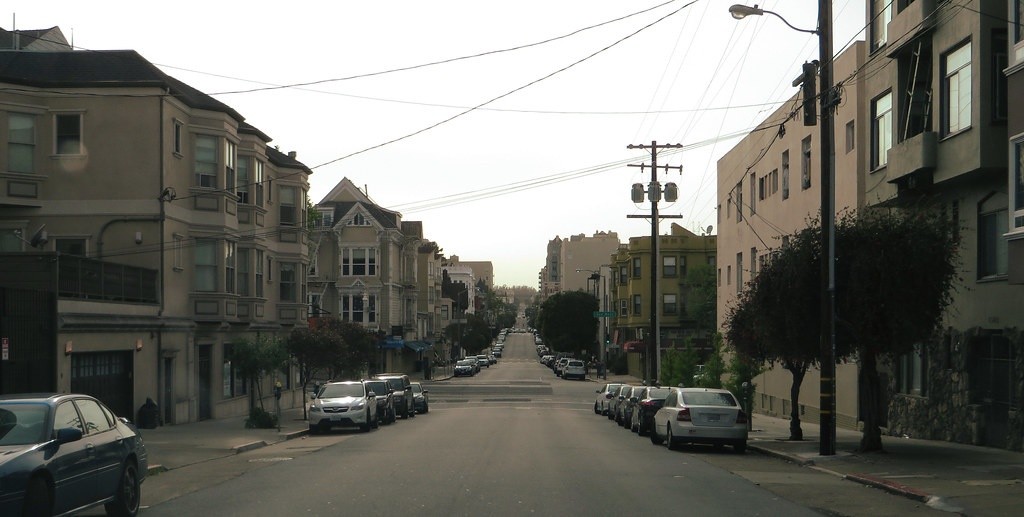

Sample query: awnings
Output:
[[405, 341, 432, 352]]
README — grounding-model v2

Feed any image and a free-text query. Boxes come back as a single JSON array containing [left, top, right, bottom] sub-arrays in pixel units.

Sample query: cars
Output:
[[608, 386, 631, 420], [619, 386, 647, 429], [453, 329, 525, 376], [651, 387, 749, 455], [630, 387, 678, 436], [410, 381, 428, 414], [526, 326, 586, 380], [364, 379, 397, 424], [0, 392, 151, 517], [594, 383, 628, 414]]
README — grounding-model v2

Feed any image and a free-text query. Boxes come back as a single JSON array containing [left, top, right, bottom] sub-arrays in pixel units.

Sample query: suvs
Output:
[[371, 372, 415, 419], [308, 379, 370, 435]]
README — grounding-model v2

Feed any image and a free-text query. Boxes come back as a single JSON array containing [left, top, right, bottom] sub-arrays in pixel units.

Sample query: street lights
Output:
[[575, 268, 608, 380], [729, 2, 841, 455]]
